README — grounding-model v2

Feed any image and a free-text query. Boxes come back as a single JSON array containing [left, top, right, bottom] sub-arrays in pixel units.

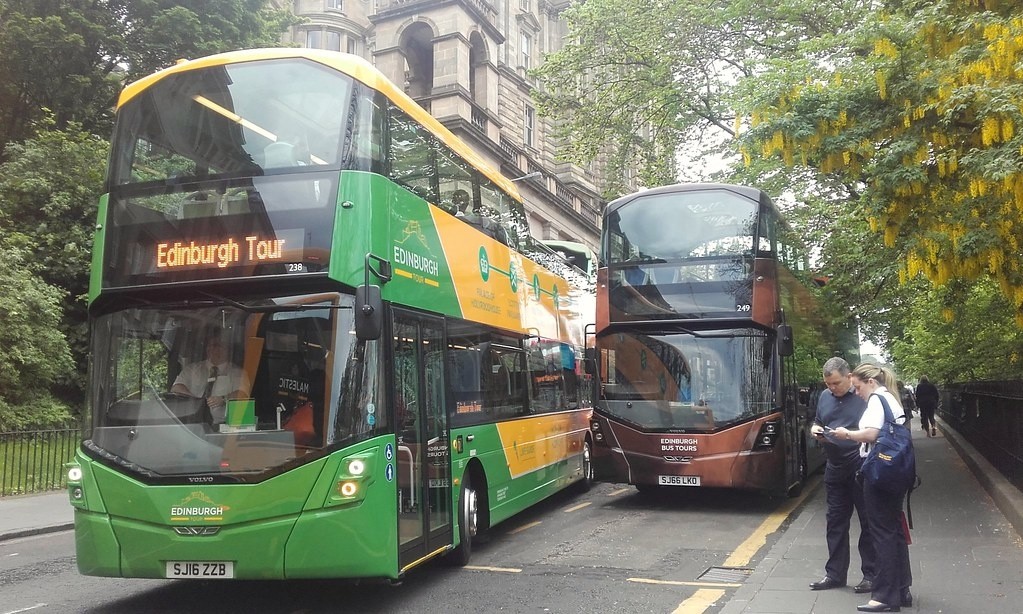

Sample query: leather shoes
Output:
[[901, 591, 912, 607], [857, 602, 900, 612], [854, 579, 873, 592], [809, 576, 846, 589]]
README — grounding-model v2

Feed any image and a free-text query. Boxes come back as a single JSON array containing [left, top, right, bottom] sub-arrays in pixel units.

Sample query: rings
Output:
[[835, 433, 838, 437]]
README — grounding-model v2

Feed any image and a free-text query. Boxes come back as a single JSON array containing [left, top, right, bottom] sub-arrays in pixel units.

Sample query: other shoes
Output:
[[927, 431, 931, 437], [932, 428, 936, 436]]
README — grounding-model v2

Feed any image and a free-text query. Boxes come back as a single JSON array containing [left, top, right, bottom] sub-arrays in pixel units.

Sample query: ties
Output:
[[201, 366, 218, 399]]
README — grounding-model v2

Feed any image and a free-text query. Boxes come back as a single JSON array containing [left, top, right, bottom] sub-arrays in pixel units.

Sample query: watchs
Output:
[[844, 430, 851, 440]]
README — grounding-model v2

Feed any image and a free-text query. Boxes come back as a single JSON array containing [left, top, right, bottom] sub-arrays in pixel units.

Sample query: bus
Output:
[[583, 182, 860, 495], [63, 40, 599, 580]]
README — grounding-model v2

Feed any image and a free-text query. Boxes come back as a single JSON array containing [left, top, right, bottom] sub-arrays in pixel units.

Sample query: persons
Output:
[[173, 333, 253, 432], [898, 375, 940, 437], [809, 357, 876, 593], [829, 363, 913, 613]]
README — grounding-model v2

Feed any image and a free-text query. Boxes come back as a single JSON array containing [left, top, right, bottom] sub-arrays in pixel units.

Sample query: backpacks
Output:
[[855, 393, 920, 530]]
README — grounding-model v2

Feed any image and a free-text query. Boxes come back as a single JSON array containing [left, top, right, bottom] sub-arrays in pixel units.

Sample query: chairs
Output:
[[617, 257, 679, 287], [264, 140, 296, 168]]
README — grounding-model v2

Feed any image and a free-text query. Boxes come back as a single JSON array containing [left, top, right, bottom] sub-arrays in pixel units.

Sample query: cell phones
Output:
[[824, 427, 834, 431], [814, 432, 825, 438]]
